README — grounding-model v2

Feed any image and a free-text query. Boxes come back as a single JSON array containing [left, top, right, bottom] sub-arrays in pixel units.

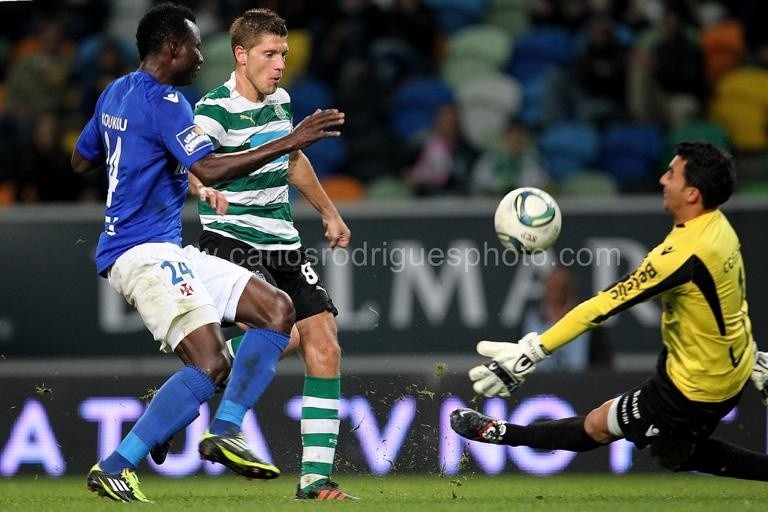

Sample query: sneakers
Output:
[[295, 477, 359, 500], [450, 407, 511, 446], [88, 460, 152, 502], [142, 389, 173, 464], [199, 429, 280, 480]]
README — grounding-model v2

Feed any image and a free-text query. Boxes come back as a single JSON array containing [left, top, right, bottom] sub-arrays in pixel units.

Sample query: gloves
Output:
[[467, 331, 552, 397], [750, 343, 768, 406]]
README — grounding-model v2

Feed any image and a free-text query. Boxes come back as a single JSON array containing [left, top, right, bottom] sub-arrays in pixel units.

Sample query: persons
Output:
[[449, 142, 768, 477], [71, 6, 344, 505], [186, 7, 360, 503]]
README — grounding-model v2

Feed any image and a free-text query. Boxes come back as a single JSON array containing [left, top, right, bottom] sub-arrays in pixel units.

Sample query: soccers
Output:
[[495, 187, 562, 255]]
